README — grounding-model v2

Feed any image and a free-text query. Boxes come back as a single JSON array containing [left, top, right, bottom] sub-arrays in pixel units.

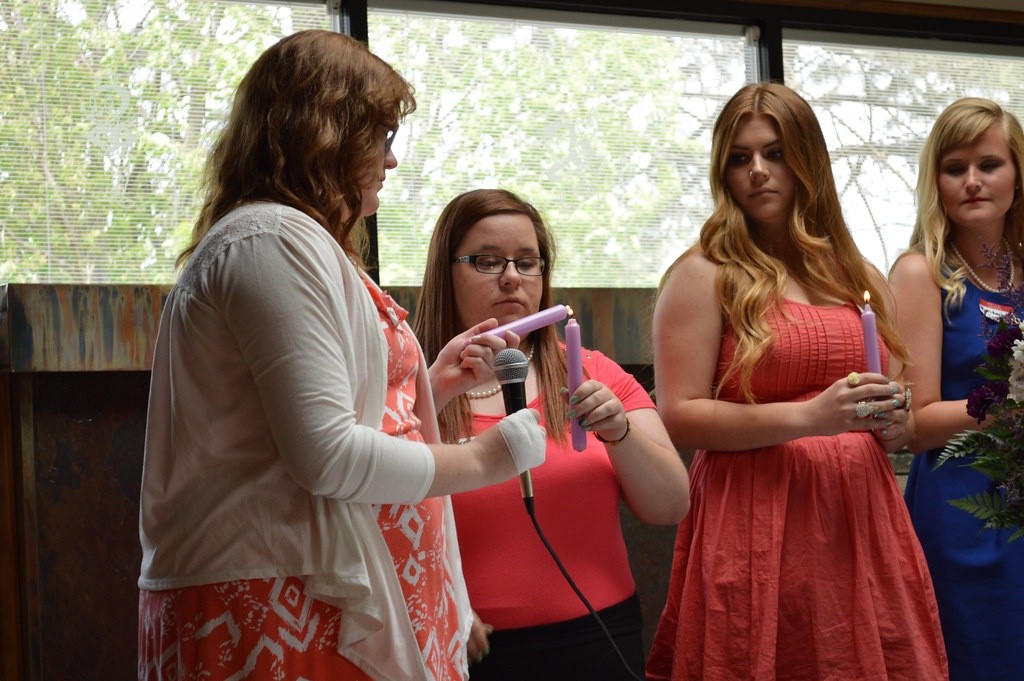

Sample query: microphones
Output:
[[493, 348, 535, 513]]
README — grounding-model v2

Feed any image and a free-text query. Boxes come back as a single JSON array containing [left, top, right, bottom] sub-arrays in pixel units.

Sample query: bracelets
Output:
[[592, 417, 631, 445]]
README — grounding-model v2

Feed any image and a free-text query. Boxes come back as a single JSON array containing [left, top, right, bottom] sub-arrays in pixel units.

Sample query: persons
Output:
[[649, 80, 953, 681], [888, 97, 1024, 681], [410, 189, 691, 681], [132, 29, 548, 681]]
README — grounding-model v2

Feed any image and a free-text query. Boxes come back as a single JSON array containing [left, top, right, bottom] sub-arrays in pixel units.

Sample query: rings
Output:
[[848, 371, 862, 386], [856, 402, 870, 417], [904, 388, 912, 411]]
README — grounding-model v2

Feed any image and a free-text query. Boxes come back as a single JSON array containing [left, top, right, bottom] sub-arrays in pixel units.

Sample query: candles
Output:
[[464, 303, 571, 347], [565, 307, 587, 452], [861, 290, 881, 373]]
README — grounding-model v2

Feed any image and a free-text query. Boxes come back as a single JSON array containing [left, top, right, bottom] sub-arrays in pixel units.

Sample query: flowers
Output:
[[966, 242, 1024, 507]]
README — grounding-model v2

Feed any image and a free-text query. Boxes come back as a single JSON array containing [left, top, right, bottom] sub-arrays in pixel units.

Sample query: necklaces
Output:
[[464, 342, 535, 398], [951, 237, 1014, 293]]
